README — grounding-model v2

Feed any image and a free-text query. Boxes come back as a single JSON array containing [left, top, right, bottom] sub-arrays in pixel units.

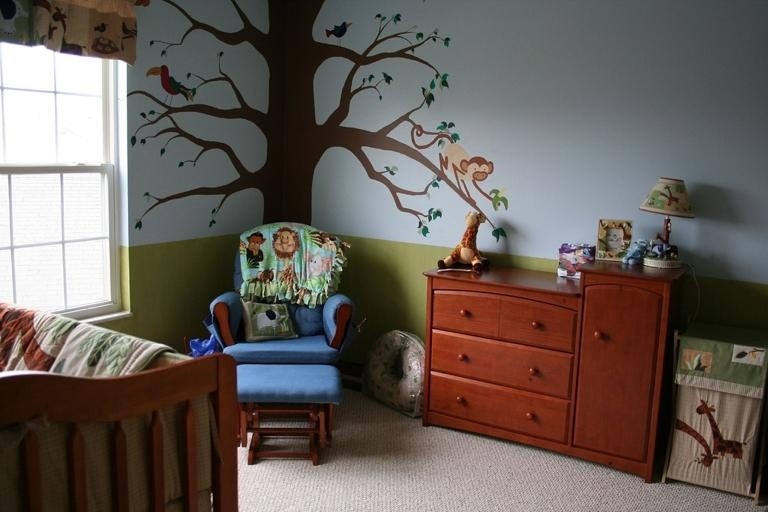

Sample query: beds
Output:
[[0, 308, 240, 511]]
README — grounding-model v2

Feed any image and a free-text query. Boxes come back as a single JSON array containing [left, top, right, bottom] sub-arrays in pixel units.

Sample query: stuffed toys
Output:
[[436, 211, 489, 274], [623, 239, 647, 266]]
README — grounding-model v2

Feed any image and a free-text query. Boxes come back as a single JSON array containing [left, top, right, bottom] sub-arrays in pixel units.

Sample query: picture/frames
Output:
[[597, 219, 632, 262]]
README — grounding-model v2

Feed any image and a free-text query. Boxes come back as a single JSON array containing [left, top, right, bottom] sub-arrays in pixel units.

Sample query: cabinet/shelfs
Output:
[[572, 258, 688, 479], [425, 261, 579, 468]]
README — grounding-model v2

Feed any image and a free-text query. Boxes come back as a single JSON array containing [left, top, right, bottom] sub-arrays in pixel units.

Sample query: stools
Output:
[[233, 364, 342, 448]]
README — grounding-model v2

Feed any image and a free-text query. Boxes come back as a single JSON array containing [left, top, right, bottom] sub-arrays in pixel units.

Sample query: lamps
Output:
[[639, 177, 697, 269]]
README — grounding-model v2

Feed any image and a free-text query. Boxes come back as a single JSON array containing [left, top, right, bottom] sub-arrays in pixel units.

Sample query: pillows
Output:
[[241, 301, 298, 341]]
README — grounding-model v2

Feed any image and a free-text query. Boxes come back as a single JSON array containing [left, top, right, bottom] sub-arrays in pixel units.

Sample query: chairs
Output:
[[206, 222, 356, 466]]
